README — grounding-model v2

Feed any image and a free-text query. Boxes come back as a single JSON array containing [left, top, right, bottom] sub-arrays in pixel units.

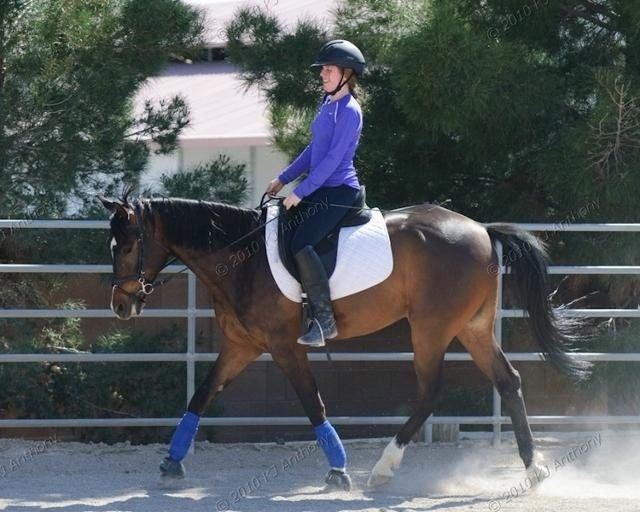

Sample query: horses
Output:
[[94, 178, 607, 492]]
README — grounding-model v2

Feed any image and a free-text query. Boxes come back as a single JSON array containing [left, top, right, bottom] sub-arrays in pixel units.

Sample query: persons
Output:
[[265, 37, 367, 347]]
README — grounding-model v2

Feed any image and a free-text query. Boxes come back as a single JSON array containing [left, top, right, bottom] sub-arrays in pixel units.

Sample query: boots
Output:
[[293, 244, 338, 345]]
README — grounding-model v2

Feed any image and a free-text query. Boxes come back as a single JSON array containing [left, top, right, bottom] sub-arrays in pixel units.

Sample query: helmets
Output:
[[311, 39, 366, 68]]
